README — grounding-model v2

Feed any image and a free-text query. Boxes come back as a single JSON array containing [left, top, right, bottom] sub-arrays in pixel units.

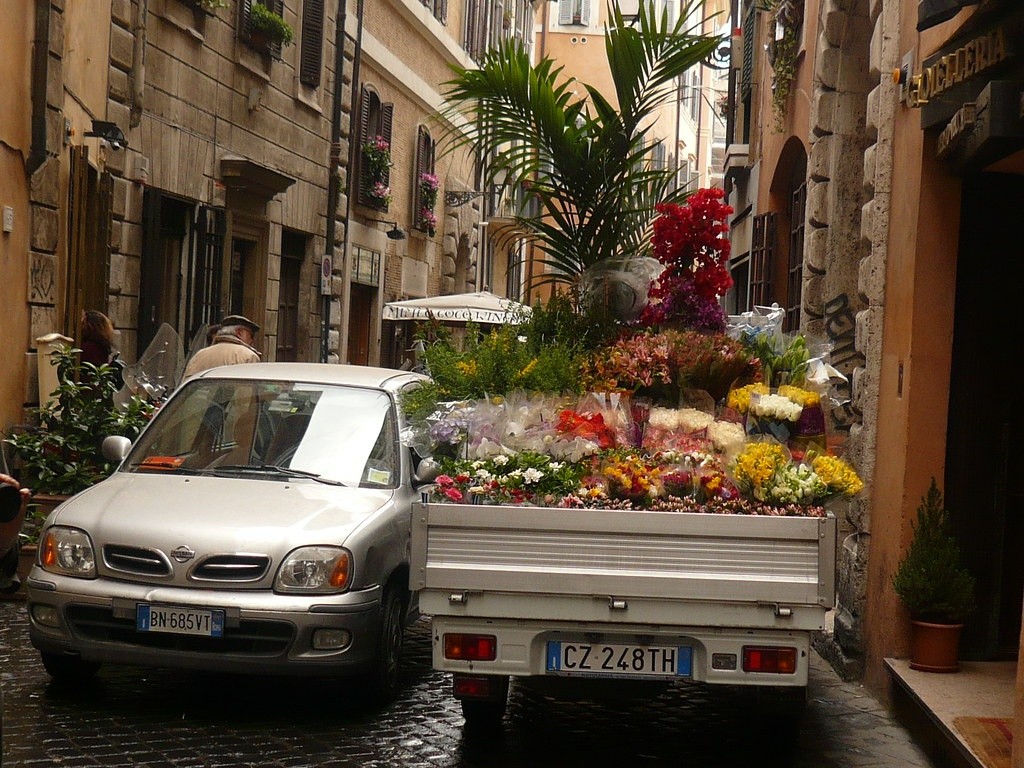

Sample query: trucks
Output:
[[405, 310, 839, 727]]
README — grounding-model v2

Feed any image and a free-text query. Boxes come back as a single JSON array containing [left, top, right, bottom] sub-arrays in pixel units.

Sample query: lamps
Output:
[[354, 212, 407, 240], [64, 119, 128, 147]]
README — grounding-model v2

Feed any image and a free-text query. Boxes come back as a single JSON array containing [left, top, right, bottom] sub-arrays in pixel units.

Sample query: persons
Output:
[[54, 308, 120, 412], [177, 314, 280, 470]]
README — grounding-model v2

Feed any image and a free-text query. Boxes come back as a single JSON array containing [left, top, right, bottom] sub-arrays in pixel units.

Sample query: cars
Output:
[[23, 362, 447, 699]]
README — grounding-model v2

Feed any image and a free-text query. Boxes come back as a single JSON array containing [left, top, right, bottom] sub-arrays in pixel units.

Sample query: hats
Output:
[[222, 315, 260, 332]]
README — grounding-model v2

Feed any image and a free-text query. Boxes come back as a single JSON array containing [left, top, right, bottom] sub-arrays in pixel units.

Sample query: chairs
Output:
[[209, 410, 313, 474]]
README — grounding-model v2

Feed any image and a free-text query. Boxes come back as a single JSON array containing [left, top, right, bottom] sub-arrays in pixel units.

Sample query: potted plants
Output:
[[502, 6, 517, 30], [247, 3, 293, 52], [17, 509, 50, 577], [5, 345, 154, 529], [893, 477, 990, 671]]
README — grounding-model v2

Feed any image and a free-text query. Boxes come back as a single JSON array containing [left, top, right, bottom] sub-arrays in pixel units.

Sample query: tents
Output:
[[384, 290, 536, 325]]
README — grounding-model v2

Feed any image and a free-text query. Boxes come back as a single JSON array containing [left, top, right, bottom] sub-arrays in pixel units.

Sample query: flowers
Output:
[[362, 131, 865, 516]]
